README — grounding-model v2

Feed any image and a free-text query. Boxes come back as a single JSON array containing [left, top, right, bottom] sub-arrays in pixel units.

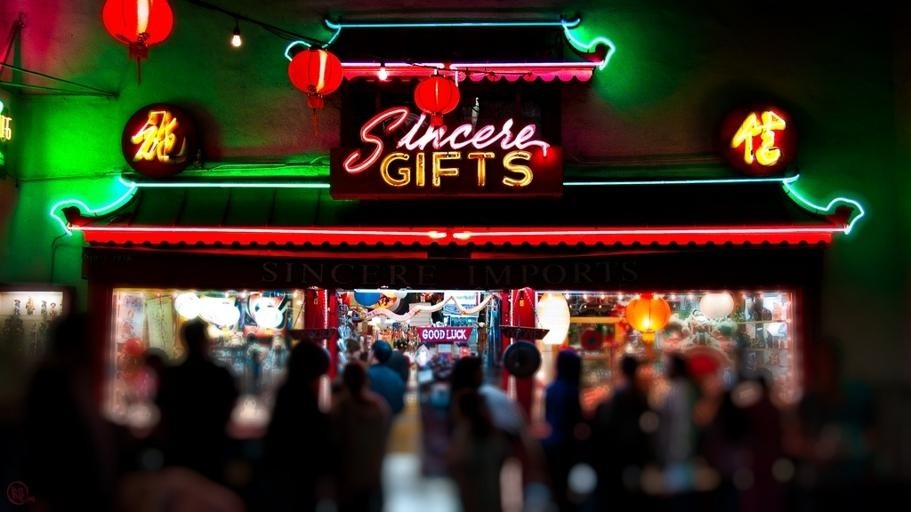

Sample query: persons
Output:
[[0, 318, 911, 512]]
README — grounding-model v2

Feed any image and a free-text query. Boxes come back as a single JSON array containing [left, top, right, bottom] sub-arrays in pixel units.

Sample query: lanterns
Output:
[[414, 75, 460, 126], [289, 49, 344, 109], [102, 0, 174, 60]]
